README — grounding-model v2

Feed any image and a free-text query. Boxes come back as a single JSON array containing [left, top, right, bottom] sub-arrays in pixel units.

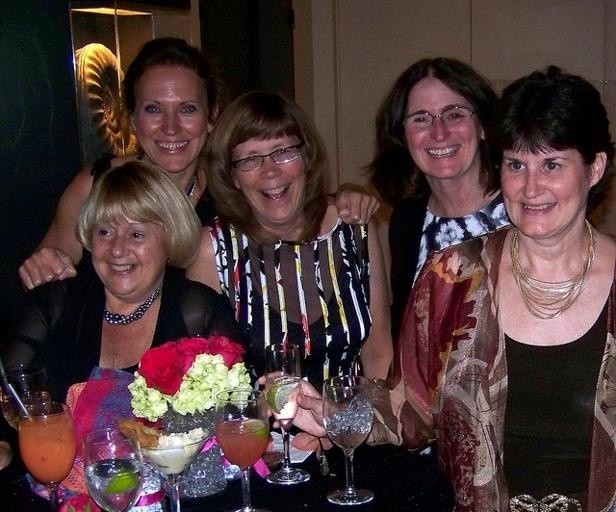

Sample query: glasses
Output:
[[230, 142, 305, 172], [403, 104, 477, 130]]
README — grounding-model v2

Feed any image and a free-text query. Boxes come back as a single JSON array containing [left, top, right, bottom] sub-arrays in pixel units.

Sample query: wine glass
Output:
[[261, 342, 312, 487], [213, 388, 268, 512], [15, 398, 78, 511], [129, 427, 212, 512], [322, 374, 376, 505]]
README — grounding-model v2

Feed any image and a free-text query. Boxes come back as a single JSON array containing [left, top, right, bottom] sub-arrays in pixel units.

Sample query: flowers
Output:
[[121, 331, 256, 425]]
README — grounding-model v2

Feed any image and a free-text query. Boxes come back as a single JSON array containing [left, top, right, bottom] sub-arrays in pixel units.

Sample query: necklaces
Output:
[[103, 283, 162, 326], [188, 177, 197, 201], [510, 220, 597, 320]]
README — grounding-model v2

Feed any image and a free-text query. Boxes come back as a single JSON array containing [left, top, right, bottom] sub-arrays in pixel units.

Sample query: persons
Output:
[[0, 161, 253, 411], [257, 66, 616, 512], [364, 58, 511, 364], [186, 90, 394, 403], [17, 37, 381, 291]]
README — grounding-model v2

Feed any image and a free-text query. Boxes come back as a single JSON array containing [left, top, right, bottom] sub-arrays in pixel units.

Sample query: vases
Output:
[[153, 400, 229, 498]]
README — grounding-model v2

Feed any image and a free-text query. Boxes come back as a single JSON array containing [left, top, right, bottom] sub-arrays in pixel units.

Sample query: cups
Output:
[[0, 360, 52, 430], [81, 424, 146, 512]]
